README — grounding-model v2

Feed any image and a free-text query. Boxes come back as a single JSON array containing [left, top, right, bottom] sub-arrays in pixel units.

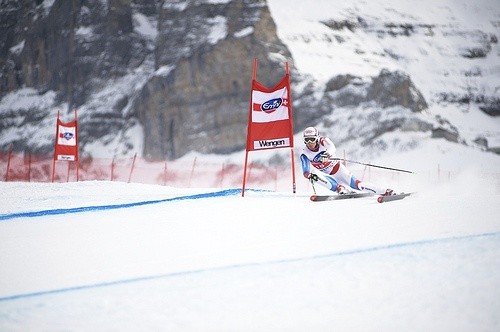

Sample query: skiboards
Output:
[[377, 192, 414, 203], [310, 192, 377, 202]]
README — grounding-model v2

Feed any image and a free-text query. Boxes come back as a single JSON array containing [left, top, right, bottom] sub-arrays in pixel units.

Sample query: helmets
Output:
[[304, 127, 320, 144]]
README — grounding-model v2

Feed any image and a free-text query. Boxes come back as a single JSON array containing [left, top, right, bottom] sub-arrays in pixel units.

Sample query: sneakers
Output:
[[382, 188, 393, 196], [336, 184, 345, 194]]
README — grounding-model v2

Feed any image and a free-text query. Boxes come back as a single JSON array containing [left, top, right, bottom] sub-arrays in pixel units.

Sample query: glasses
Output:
[[304, 134, 320, 143]]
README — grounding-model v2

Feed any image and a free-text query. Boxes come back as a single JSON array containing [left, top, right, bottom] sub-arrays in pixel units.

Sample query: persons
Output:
[[300, 127, 396, 203]]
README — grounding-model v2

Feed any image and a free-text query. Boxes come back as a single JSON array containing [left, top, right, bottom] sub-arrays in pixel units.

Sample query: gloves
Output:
[[317, 154, 329, 164], [308, 173, 319, 183]]
